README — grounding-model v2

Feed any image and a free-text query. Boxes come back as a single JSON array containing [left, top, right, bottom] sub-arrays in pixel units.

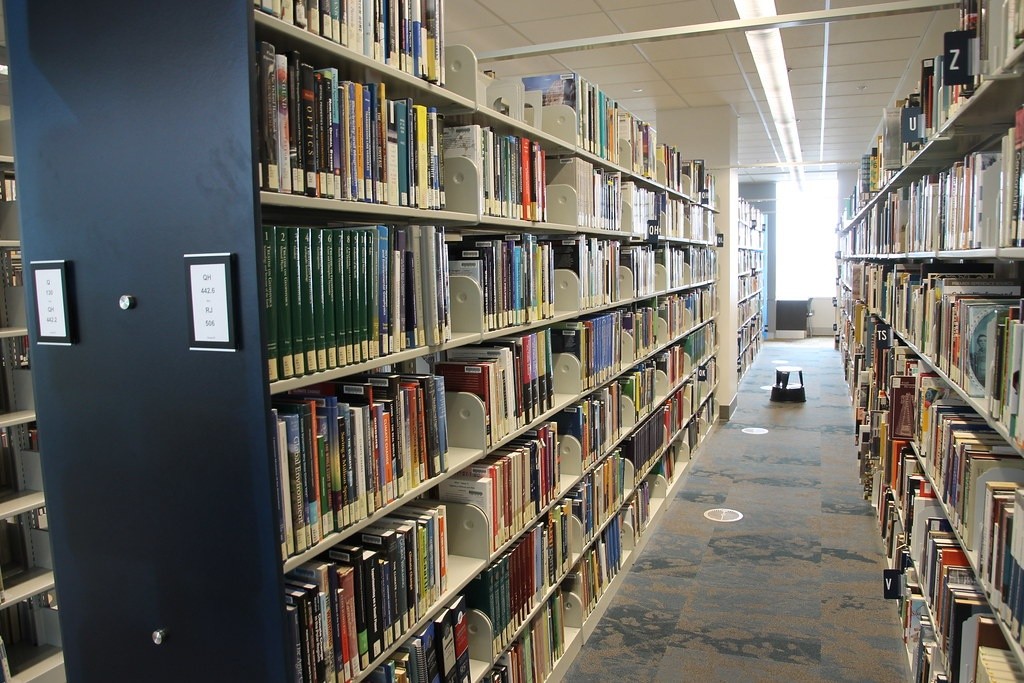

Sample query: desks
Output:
[[776, 300, 808, 330]]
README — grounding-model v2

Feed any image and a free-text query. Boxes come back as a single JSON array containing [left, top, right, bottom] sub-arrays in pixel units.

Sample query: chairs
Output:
[[806, 298, 813, 338]]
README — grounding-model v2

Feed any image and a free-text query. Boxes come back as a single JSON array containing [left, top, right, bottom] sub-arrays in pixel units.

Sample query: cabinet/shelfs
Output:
[[837, 0, 1024, 683], [0, 0, 765, 683]]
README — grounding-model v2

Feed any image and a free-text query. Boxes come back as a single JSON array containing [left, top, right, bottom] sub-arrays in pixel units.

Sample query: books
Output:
[[831, 0, 1024, 683], [247, 0, 718, 683], [735, 195, 768, 383]]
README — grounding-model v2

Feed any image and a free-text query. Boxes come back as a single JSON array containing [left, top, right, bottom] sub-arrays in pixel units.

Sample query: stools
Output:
[[770, 367, 806, 403]]
